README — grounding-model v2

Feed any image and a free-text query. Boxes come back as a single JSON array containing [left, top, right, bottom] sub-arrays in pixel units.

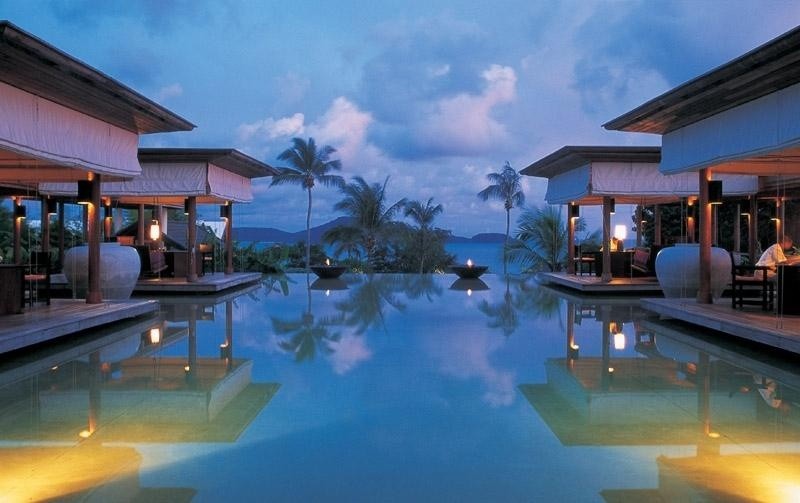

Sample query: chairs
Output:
[[575, 243, 595, 275], [731, 249, 775, 310], [200, 243, 216, 274], [24, 251, 50, 305]]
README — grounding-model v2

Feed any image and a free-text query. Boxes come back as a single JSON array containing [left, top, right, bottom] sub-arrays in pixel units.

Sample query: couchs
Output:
[[117, 243, 168, 278]]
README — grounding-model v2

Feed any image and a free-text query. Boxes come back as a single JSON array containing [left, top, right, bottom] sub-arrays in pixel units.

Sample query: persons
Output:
[[600, 234, 620, 252], [753, 231, 800, 285]]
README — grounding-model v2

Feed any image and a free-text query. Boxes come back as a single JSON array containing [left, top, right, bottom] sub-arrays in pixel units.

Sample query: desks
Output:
[[591, 246, 633, 278], [0, 262, 26, 315], [161, 249, 189, 278], [777, 258, 800, 315]]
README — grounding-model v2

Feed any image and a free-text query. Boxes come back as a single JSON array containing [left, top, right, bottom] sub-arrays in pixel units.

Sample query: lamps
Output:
[[613, 224, 628, 249], [612, 321, 626, 349], [150, 325, 161, 343], [150, 224, 160, 241]]
